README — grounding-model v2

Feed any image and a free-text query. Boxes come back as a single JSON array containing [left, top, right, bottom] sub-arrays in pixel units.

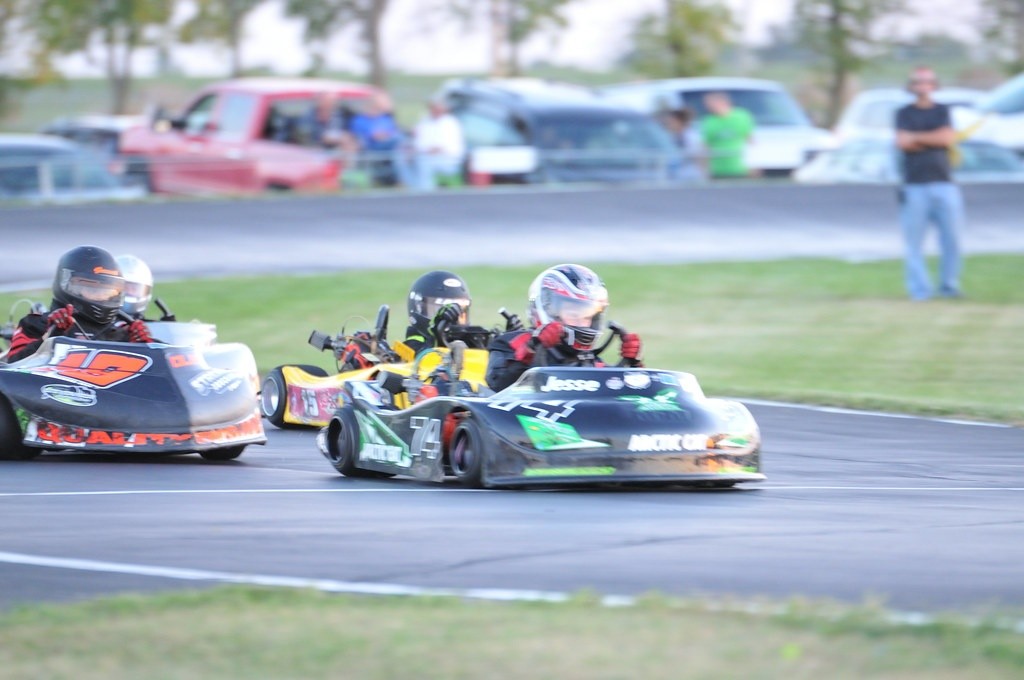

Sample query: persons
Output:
[[6, 245, 177, 364], [338, 88, 471, 189], [693, 92, 763, 182], [390, 263, 645, 394], [889, 65, 967, 304]]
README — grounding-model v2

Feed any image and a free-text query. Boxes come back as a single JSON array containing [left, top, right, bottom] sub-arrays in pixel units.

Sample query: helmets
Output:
[[408, 271, 471, 340], [111, 255, 153, 313], [52, 246, 123, 325], [528, 264, 610, 361]]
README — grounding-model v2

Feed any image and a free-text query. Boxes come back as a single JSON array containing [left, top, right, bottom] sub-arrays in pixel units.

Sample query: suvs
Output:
[[430, 76, 837, 182]]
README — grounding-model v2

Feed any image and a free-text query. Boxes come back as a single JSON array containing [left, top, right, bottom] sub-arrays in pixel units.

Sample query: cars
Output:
[[0, 75, 398, 196], [789, 67, 1024, 182]]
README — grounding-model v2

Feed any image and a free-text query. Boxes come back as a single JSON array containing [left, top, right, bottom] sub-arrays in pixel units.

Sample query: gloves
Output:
[[49, 302, 75, 333], [429, 304, 462, 342], [508, 314, 524, 334], [622, 334, 643, 364], [530, 322, 563, 349], [129, 319, 152, 341]]
